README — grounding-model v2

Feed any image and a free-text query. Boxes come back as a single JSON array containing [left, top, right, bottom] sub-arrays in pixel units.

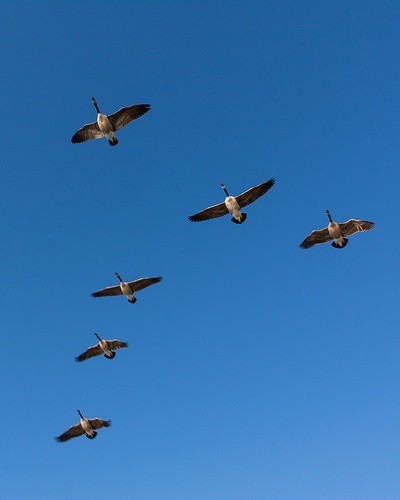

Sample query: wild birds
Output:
[[298, 210, 376, 249], [89, 272, 162, 304], [71, 97, 152, 147], [187, 176, 276, 225], [54, 409, 112, 442], [73, 332, 128, 363]]
